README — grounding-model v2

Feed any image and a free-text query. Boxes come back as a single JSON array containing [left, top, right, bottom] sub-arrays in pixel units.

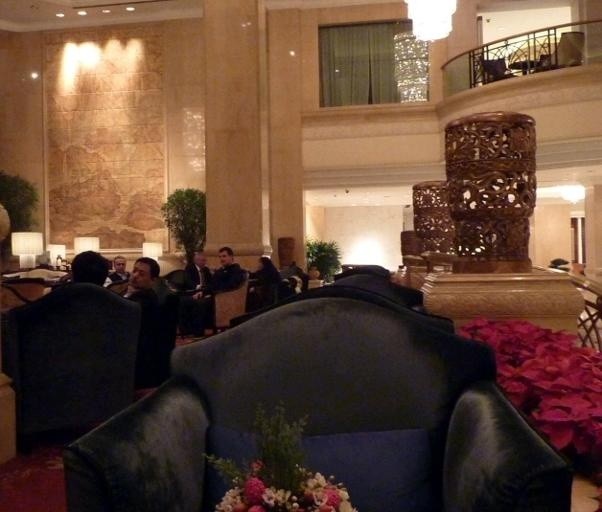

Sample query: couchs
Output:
[[1, 270, 455, 464], [63, 297, 573, 512]]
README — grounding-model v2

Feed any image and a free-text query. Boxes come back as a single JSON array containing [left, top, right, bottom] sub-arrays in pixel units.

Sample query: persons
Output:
[[52, 247, 279, 337]]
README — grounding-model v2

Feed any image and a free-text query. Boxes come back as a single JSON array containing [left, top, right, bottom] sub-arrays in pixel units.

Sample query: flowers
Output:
[[199, 401, 357, 512], [459, 312, 602, 472]]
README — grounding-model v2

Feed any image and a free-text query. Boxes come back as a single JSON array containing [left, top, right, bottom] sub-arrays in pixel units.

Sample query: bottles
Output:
[[56, 255, 62, 266]]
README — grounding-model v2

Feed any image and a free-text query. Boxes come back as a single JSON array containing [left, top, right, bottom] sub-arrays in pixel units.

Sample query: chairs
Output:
[[477, 29, 585, 84]]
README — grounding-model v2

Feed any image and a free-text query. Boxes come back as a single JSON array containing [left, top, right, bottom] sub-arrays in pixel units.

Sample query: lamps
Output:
[[11, 230, 102, 269]]
